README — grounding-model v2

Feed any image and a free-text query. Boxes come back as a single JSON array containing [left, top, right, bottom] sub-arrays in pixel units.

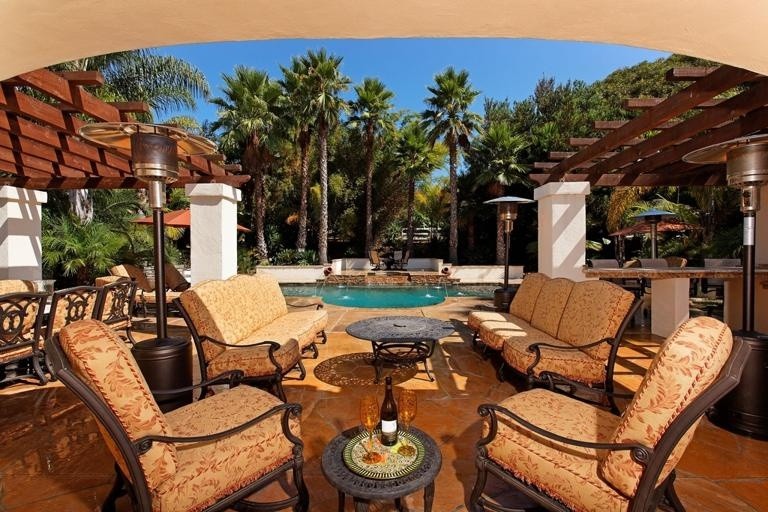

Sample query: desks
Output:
[[583, 263, 768, 338]]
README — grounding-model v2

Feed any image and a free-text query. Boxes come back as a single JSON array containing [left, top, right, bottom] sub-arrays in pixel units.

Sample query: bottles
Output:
[[379, 375, 398, 447]]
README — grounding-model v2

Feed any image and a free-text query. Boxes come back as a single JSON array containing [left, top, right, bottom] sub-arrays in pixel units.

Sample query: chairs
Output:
[[0, 279, 39, 294], [105, 263, 153, 291], [44, 282, 104, 384], [95, 275, 184, 317], [156, 261, 190, 292], [92, 277, 136, 344], [590, 255, 741, 301], [364, 243, 412, 271], [1, 291, 47, 385], [45, 318, 311, 512], [471, 317, 751, 511]]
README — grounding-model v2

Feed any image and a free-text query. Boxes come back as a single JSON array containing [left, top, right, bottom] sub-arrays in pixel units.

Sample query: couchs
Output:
[[469, 271, 643, 417], [173, 272, 330, 403]]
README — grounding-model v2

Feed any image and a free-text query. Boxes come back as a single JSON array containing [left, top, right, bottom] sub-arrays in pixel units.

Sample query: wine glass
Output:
[[396, 390, 419, 458], [358, 394, 383, 466]]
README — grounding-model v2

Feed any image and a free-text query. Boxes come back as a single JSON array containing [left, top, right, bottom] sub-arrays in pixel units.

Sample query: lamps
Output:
[[74, 119, 221, 415], [629, 205, 678, 267], [682, 127, 768, 442], [481, 193, 535, 311]]
